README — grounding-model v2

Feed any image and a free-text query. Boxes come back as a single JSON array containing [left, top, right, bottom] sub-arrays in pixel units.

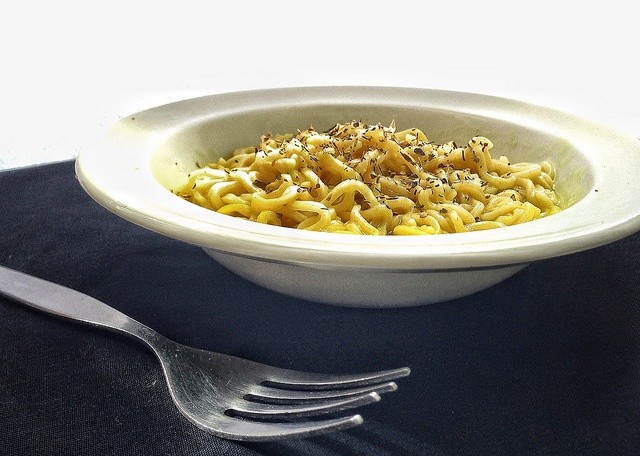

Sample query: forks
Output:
[[0, 264, 411, 442]]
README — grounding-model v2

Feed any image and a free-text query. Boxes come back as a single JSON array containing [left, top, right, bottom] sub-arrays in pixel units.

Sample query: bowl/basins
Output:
[[75, 84, 639, 308]]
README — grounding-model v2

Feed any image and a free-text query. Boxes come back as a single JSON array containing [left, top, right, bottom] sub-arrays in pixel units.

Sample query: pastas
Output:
[[177, 116, 559, 234]]
